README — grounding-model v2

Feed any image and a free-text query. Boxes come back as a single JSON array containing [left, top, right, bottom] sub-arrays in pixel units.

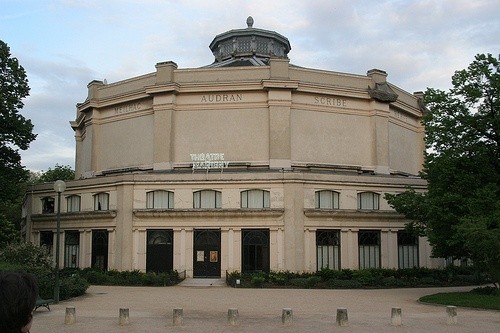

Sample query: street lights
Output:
[[53, 180, 66, 269]]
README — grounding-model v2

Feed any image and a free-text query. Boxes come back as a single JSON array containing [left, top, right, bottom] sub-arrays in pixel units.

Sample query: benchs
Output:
[[32, 293, 55, 313]]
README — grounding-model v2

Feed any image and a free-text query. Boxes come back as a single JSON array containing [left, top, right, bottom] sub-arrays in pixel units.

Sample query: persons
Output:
[[0, 269, 41, 333]]
